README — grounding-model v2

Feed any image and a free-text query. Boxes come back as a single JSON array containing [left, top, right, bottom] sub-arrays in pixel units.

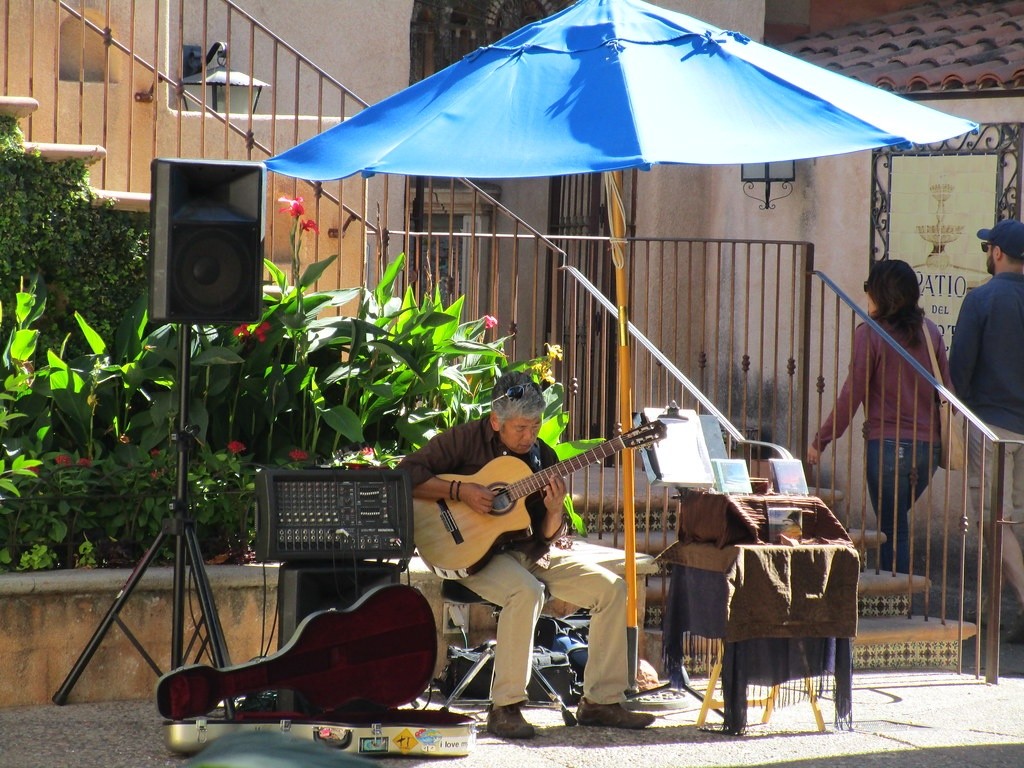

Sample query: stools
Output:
[[440, 579, 577, 728]]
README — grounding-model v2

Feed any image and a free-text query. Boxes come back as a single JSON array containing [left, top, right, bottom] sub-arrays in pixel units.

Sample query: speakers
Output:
[[149, 158, 267, 324], [278, 561, 400, 651]]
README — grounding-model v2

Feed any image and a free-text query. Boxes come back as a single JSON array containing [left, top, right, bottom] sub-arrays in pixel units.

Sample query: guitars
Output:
[[413, 417, 669, 582]]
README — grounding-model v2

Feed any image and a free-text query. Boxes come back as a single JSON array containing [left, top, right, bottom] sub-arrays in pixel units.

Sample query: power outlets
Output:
[[443, 602, 469, 634]]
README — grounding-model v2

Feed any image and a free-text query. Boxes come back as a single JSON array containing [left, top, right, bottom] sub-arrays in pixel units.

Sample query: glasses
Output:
[[492, 382, 542, 408], [981, 242, 994, 252], [864, 281, 869, 292]]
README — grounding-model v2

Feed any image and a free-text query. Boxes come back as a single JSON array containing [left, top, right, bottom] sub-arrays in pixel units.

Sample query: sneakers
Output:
[[486, 703, 535, 739], [577, 696, 655, 729]]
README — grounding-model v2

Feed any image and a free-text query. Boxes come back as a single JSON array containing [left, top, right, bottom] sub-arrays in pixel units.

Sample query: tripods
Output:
[[53, 324, 232, 725]]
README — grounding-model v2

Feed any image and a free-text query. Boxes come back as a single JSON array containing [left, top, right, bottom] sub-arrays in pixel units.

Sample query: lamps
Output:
[[739, 160, 795, 209], [182, 40, 272, 114]]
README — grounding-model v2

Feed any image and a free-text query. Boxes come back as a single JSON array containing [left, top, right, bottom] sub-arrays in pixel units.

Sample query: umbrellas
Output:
[[266, 0, 979, 698]]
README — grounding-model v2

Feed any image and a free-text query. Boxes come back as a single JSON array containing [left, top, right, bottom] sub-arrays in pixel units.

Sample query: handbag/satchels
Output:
[[442, 608, 592, 703], [939, 400, 963, 471]]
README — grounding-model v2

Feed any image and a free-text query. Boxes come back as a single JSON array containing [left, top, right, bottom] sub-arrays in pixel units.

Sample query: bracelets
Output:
[[457, 481, 461, 501], [450, 480, 455, 500]]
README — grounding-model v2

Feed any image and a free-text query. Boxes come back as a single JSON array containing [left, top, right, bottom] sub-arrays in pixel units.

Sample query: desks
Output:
[[651, 541, 861, 734]]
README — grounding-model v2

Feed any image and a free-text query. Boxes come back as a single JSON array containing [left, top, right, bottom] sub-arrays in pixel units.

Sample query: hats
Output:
[[636, 659, 670, 693], [977, 219, 1024, 260]]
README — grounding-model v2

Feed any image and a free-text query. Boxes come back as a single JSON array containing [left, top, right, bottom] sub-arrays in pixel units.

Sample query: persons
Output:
[[809, 259, 956, 574], [397, 371, 655, 739], [949, 220, 1024, 642]]
